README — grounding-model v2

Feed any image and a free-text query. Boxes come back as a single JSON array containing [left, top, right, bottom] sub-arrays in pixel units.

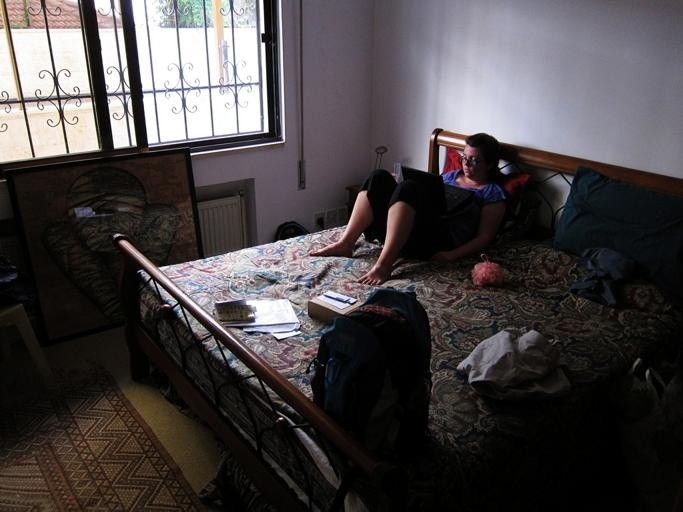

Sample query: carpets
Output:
[[0, 359, 208, 512]]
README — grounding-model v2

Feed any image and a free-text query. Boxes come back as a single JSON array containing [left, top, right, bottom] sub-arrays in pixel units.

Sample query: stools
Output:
[[0, 303, 61, 393]]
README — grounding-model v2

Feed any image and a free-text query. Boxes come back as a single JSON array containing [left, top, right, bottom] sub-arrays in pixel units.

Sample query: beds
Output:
[[111, 130, 682, 512]]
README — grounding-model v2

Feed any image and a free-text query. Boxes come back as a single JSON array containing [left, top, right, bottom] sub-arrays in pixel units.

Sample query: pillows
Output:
[[553, 165, 682, 285], [438, 145, 542, 247]]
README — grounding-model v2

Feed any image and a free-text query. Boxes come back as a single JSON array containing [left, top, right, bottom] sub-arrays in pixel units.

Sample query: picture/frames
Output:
[[3, 146, 204, 346]]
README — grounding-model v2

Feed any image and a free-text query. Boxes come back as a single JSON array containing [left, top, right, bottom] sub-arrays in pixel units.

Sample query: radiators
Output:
[[197, 195, 247, 258]]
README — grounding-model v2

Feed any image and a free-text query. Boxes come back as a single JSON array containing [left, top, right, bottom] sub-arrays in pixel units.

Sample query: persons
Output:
[[309, 133, 507, 288]]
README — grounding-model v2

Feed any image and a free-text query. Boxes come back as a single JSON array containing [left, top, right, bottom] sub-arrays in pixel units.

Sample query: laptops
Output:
[[401, 165, 475, 216]]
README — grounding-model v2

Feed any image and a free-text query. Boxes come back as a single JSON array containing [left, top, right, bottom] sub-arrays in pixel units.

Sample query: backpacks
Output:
[[305, 285, 433, 481]]
